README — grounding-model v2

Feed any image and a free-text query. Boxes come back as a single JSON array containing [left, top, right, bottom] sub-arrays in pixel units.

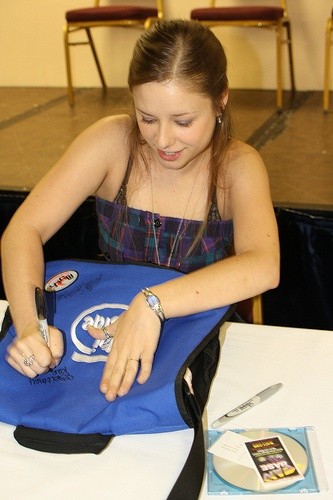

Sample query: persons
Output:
[[0, 20, 281, 400]]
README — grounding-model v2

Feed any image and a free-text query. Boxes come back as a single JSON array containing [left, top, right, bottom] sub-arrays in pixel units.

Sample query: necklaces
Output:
[[147, 150, 204, 268]]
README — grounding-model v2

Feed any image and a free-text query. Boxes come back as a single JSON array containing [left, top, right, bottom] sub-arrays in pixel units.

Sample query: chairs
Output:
[[322, 8, 333, 114], [63, 0, 162, 108], [190, 0, 299, 108]]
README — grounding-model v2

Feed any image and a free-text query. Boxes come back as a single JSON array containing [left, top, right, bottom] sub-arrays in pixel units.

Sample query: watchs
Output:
[[141, 288, 165, 323]]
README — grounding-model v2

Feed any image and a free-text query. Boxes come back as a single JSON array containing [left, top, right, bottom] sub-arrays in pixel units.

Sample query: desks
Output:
[[0, 299, 333, 500]]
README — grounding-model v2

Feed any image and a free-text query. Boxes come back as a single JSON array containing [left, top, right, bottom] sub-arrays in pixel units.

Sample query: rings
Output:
[[128, 358, 140, 363], [102, 325, 111, 340], [23, 355, 36, 367]]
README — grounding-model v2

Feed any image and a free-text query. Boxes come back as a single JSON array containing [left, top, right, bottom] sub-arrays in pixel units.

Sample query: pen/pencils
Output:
[[34, 285, 50, 351], [210, 382, 283, 429]]
